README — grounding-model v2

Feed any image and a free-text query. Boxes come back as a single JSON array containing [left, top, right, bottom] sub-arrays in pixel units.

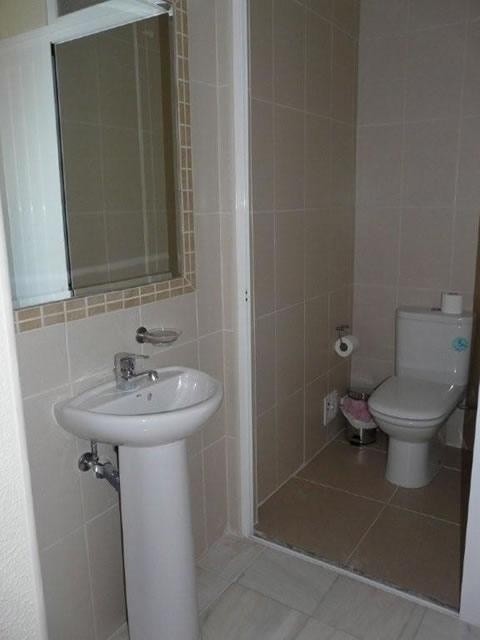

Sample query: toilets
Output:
[[367, 305, 474, 489]]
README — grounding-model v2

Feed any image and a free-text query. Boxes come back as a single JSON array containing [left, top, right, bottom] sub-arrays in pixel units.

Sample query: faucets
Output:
[[114, 352, 159, 390]]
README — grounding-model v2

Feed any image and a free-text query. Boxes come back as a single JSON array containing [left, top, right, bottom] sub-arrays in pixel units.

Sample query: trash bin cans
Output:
[[345, 391, 379, 445]]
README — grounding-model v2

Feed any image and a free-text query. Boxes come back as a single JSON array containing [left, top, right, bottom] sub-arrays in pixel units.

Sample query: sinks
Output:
[[54, 366, 225, 447]]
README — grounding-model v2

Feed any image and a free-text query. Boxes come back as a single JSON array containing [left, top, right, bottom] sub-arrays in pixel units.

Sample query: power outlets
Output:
[[322, 389, 339, 426]]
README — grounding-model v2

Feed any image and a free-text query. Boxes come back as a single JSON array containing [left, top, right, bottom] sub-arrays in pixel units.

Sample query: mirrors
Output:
[[0, 0, 188, 314]]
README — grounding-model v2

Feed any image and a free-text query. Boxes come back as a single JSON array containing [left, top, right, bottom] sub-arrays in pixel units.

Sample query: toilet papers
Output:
[[441, 292, 463, 314], [334, 335, 359, 358]]
